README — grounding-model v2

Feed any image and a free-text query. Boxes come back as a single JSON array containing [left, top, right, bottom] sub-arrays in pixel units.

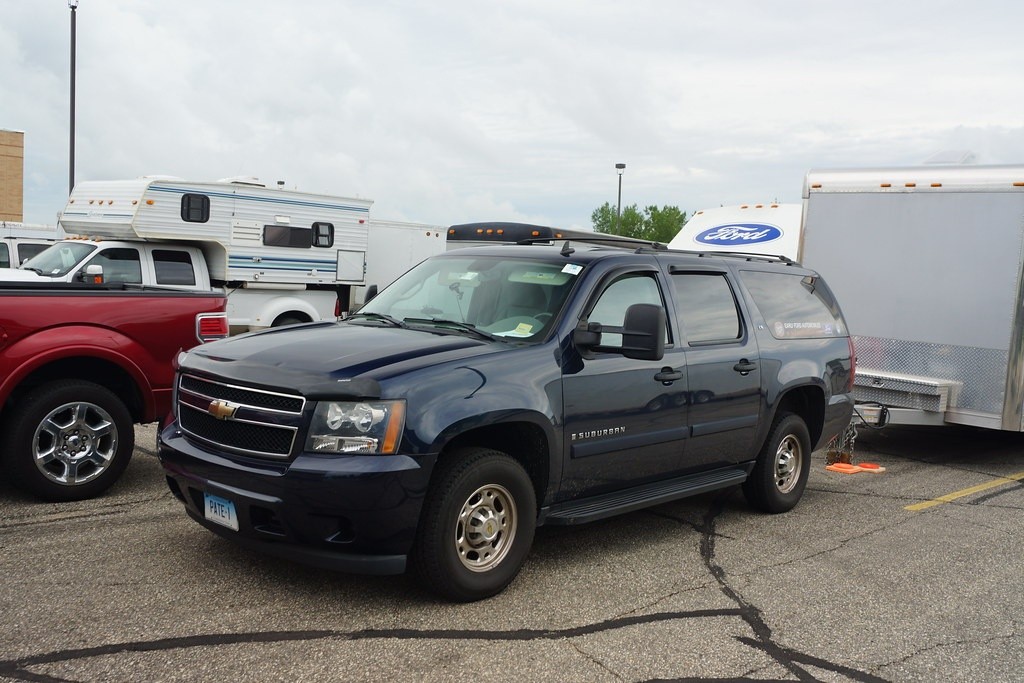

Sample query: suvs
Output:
[[157, 236, 857, 603]]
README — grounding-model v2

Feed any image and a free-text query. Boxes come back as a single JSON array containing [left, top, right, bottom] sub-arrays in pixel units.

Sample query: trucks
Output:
[[0, 176, 374, 335]]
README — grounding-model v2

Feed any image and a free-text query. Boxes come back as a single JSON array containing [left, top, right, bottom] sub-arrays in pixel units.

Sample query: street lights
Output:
[[614, 163, 626, 235], [67, 0, 78, 200]]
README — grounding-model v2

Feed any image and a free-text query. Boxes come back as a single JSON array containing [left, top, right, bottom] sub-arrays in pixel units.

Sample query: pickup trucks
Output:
[[0, 282, 228, 503]]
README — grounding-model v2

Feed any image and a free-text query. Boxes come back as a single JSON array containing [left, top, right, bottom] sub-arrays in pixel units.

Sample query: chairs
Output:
[[490, 283, 545, 323]]
